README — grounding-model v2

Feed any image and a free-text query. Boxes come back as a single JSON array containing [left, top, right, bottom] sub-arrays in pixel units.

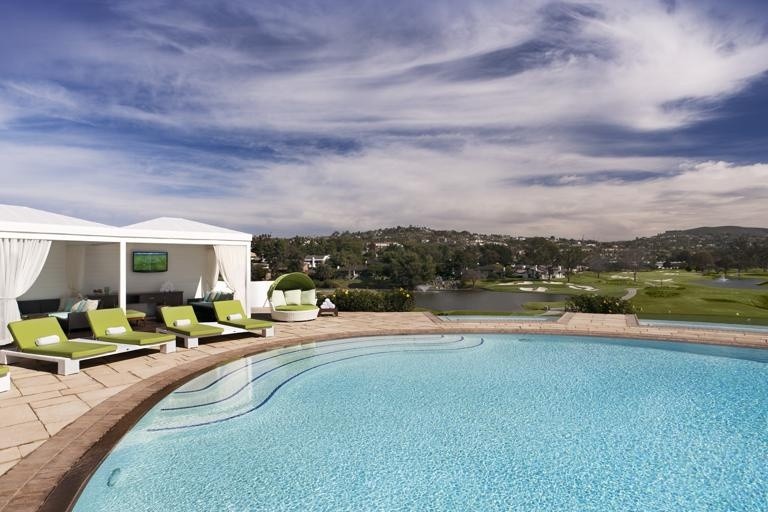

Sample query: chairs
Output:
[[1, 298, 274, 392]]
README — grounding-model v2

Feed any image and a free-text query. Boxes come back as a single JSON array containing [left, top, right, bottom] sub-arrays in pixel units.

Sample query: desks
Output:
[[318, 309, 338, 317]]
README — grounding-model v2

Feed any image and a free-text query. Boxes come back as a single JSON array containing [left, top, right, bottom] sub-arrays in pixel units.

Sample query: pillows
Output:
[[270, 290, 287, 306], [204, 292, 234, 302], [301, 289, 316, 306], [284, 289, 301, 305]]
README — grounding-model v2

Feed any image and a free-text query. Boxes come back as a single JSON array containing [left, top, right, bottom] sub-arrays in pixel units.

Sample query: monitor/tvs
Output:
[[132, 251, 168, 272]]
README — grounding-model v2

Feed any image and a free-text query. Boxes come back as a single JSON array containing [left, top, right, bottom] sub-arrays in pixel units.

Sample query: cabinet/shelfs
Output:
[[138, 291, 183, 306]]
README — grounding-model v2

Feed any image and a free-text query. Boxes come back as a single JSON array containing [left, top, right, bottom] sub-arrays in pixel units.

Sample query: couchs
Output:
[[267, 272, 320, 322]]
